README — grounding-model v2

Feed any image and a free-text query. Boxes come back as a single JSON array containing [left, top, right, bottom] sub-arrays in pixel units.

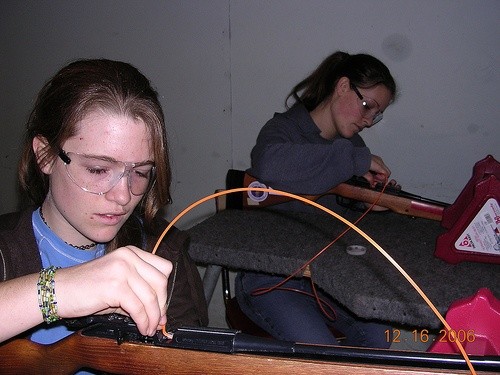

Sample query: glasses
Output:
[[353, 85, 383, 125], [53, 143, 158, 196]]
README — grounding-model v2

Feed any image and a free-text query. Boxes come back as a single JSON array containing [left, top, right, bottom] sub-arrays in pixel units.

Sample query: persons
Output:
[[234, 51, 401, 350], [0, 60, 209, 344]]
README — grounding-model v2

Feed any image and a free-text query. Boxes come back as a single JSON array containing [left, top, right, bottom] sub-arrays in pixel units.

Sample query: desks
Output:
[[184, 209, 500, 330]]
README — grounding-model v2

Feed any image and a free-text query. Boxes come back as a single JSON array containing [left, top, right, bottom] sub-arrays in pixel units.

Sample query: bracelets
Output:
[[37, 266, 62, 325]]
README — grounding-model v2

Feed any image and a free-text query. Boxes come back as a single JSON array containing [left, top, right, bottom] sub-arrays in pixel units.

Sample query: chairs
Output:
[[214, 188, 271, 337]]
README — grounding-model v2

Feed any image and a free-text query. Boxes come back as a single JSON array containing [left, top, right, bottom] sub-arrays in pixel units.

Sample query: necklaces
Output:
[[39, 206, 96, 250]]
